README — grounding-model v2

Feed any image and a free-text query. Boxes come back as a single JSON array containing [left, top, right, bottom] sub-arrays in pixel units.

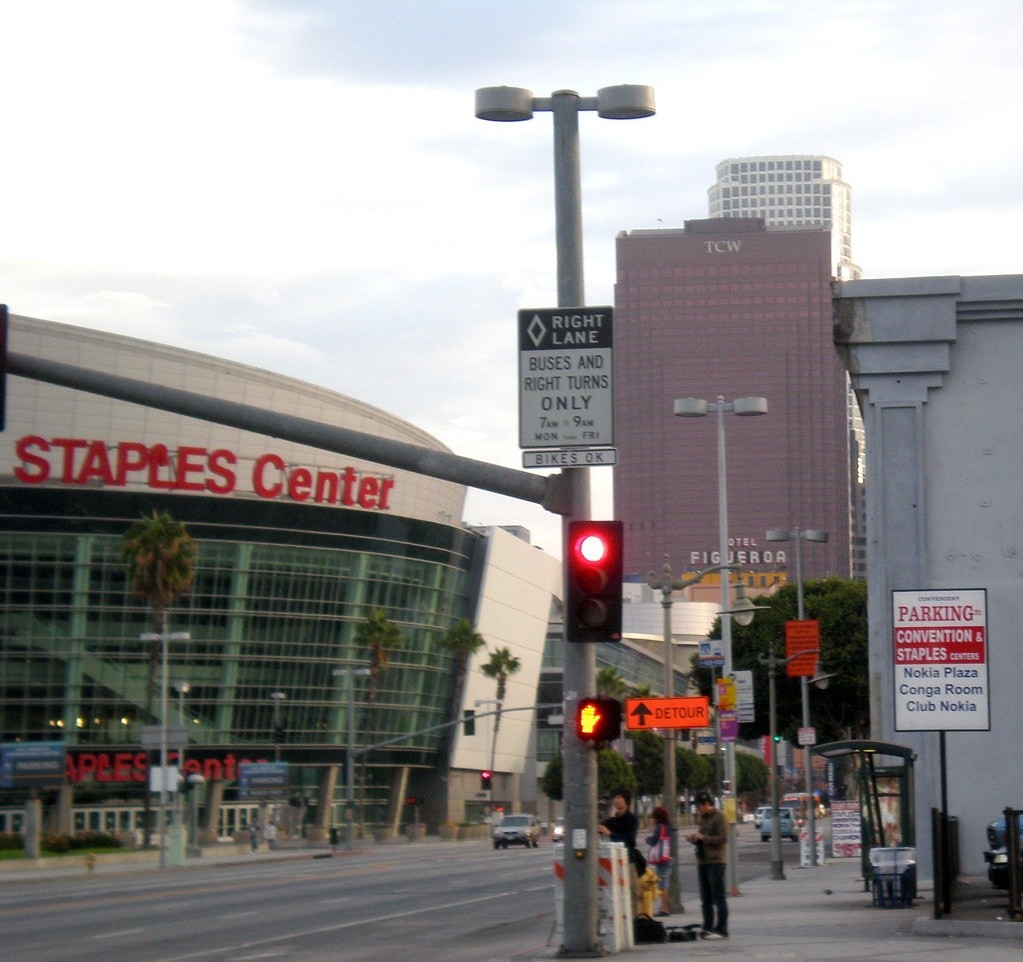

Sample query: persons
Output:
[[682, 791, 729, 939], [248, 821, 257, 852], [645, 807, 675, 917], [263, 821, 276, 851], [598, 788, 639, 920]]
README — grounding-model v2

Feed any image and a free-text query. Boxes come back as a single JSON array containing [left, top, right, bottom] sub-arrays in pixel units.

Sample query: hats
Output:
[[689, 790, 715, 804]]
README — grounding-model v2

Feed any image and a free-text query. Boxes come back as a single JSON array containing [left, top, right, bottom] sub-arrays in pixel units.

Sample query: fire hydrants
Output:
[[636, 866, 664, 917]]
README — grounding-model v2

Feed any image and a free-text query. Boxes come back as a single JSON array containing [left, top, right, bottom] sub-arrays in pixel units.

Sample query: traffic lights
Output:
[[566, 518, 623, 644], [481, 770, 492, 790], [575, 698, 620, 741]]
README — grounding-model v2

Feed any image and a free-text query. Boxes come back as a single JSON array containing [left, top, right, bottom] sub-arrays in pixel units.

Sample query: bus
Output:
[[780, 793, 821, 820]]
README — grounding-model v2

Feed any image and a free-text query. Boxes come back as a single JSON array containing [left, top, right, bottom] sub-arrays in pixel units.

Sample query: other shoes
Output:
[[698, 929, 729, 939]]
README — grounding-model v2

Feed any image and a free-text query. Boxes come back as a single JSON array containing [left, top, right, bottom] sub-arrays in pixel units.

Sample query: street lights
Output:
[[172, 681, 191, 746], [138, 629, 193, 867], [764, 526, 830, 866], [645, 552, 773, 913], [671, 394, 769, 900], [756, 648, 838, 880], [270, 691, 286, 762], [330, 668, 371, 851], [474, 698, 503, 816], [473, 84, 654, 958]]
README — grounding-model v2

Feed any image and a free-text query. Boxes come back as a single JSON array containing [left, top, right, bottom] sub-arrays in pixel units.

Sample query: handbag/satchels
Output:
[[636, 912, 666, 944], [648, 824, 672, 864]]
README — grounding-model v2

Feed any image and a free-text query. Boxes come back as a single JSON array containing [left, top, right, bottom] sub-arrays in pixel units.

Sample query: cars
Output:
[[491, 813, 539, 849], [754, 806, 769, 828], [551, 815, 565, 841], [984, 819, 1023, 889], [760, 807, 801, 842]]
